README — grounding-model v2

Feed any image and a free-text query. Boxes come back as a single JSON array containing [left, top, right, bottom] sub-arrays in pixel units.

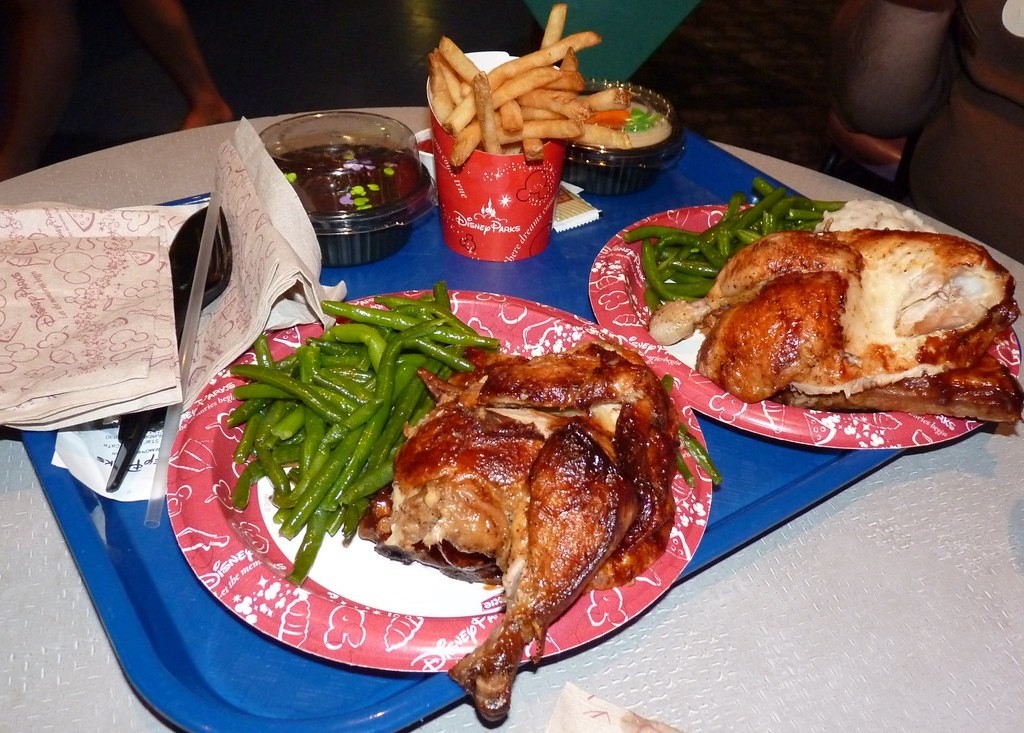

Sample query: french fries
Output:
[[427, 5, 632, 168]]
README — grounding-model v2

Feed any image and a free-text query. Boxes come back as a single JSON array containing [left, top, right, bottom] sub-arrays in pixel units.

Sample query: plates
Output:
[[167, 290, 712, 672], [588, 205, 1020, 449]]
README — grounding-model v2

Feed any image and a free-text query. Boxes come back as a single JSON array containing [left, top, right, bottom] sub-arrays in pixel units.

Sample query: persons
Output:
[[0, 0, 234, 184], [823, 0, 1024, 267]]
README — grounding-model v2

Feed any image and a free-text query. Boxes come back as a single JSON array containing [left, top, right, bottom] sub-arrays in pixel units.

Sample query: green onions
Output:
[[622, 108, 662, 131]]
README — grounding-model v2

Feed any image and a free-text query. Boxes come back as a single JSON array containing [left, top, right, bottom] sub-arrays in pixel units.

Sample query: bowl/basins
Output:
[[561, 104, 688, 198], [406, 126, 435, 179], [254, 144, 436, 269]]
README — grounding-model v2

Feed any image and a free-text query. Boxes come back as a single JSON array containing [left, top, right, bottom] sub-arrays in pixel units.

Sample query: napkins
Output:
[[0, 113, 349, 431]]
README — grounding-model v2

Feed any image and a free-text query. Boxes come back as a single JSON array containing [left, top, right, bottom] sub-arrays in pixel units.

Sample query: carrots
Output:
[[585, 108, 632, 128]]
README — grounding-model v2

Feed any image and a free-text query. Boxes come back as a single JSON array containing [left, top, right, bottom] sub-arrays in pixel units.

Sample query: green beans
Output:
[[621, 177, 850, 309], [659, 374, 723, 488], [222, 280, 501, 586]]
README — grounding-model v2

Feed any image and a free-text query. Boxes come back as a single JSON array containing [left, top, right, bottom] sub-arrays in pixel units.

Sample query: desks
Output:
[[0, 105, 1024, 733]]
